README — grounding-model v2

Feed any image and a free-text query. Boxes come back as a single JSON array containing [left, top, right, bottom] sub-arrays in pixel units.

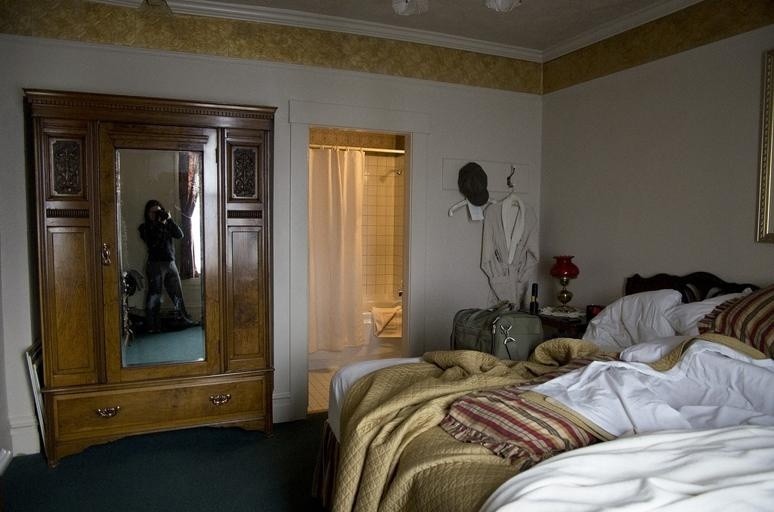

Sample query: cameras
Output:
[[156, 209, 168, 221]]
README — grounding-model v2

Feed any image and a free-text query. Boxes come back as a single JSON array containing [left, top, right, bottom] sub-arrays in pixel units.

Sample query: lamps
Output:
[[549, 253, 581, 314]]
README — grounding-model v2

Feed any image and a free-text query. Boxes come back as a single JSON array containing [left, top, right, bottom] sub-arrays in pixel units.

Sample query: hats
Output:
[[456, 161, 490, 206]]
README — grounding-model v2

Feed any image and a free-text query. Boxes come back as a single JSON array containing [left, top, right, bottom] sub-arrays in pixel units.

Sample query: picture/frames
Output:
[[753, 48, 773, 244], [22, 337, 48, 456]]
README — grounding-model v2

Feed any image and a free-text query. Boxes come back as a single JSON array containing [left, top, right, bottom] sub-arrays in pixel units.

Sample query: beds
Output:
[[306, 268, 773, 512]]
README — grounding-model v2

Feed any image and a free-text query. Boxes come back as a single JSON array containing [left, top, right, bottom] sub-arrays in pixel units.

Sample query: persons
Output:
[[139, 199, 200, 333]]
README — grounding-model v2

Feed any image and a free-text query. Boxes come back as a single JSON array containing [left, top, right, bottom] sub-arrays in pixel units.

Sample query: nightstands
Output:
[[519, 309, 596, 340]]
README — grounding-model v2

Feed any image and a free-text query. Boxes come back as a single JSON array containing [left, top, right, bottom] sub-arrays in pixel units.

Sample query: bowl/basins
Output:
[[584, 304, 606, 317]]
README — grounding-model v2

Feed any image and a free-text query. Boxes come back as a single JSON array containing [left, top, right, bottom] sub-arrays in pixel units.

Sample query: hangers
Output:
[[508, 181, 518, 208], [448, 194, 498, 217]]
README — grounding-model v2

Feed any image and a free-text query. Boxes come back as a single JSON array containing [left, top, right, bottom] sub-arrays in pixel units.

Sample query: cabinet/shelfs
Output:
[[20, 85, 280, 470]]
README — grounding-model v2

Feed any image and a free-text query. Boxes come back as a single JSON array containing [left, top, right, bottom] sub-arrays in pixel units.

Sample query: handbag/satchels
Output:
[[448, 298, 546, 362]]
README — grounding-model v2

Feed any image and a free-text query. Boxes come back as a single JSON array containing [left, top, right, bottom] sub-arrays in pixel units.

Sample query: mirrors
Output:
[[113, 145, 206, 368]]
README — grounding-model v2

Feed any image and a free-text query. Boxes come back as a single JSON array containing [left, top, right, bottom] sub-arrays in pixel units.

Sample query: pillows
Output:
[[662, 288, 753, 340]]
